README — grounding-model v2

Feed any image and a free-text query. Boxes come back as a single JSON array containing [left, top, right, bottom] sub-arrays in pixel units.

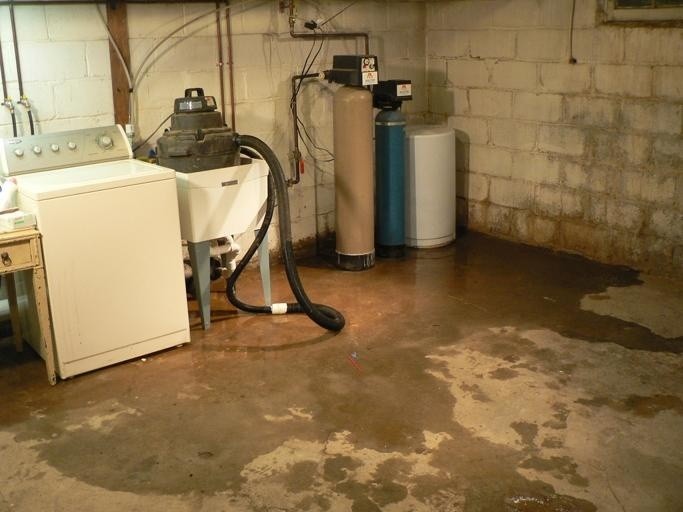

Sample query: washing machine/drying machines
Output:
[[0, 124, 191, 382]]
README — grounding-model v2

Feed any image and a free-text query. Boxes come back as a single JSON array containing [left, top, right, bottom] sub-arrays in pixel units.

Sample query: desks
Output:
[[0, 230, 57, 388]]
